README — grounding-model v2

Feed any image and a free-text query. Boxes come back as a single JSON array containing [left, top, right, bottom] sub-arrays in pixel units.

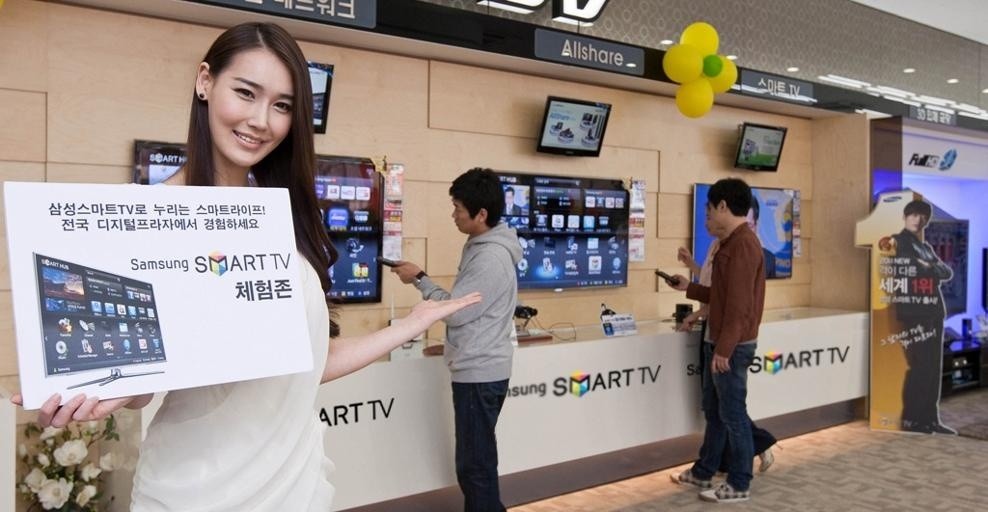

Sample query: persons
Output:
[[670, 177, 765, 503], [748, 198, 776, 278], [676, 201, 784, 475], [891, 200, 958, 437], [501, 186, 522, 216], [389, 167, 524, 511], [10, 22, 483, 510]]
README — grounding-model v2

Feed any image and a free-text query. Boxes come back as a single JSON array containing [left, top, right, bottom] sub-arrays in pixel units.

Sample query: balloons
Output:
[[663, 23, 738, 119]]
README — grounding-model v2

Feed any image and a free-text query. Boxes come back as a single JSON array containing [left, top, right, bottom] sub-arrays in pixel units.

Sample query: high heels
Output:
[[759, 429, 784, 472]]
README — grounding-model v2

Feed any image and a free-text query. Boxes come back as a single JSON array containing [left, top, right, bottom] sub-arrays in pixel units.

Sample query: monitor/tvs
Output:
[[493, 171, 629, 289], [692, 183, 793, 280], [133, 139, 384, 304], [734, 122, 787, 172], [306, 61, 334, 134], [536, 96, 612, 157], [32, 252, 168, 390]]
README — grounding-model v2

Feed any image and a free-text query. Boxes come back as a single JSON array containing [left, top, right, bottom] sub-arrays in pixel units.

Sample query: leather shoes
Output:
[[900, 419, 955, 435]]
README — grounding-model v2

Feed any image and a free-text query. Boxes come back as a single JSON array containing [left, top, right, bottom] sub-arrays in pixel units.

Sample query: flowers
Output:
[[16, 412, 123, 511]]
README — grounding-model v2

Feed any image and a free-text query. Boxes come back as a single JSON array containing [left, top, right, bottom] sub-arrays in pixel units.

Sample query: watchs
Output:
[[413, 270, 426, 286]]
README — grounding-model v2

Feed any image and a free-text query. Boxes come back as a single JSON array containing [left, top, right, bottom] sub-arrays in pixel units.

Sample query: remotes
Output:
[[376, 256, 397, 267], [655, 271, 680, 285]]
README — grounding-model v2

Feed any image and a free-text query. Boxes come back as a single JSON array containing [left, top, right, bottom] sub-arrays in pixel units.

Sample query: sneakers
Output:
[[669, 467, 711, 489], [698, 480, 750, 503]]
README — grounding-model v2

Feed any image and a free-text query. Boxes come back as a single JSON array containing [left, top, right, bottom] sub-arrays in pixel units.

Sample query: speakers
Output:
[[676, 304, 692, 321]]
